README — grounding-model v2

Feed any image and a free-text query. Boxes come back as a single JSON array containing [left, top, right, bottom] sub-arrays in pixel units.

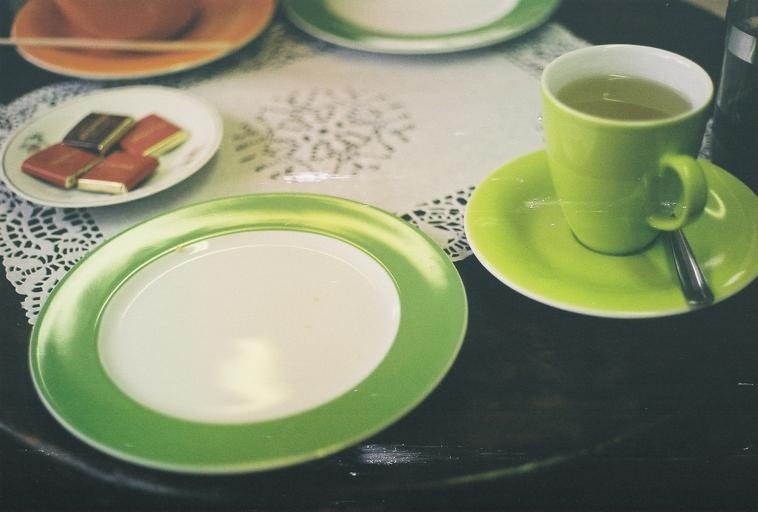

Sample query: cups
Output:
[[51, 0, 201, 44], [540, 43, 716, 256]]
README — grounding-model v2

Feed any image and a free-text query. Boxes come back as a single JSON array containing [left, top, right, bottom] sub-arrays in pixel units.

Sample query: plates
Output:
[[279, 0, 563, 55], [0, 78, 226, 209], [7, 0, 280, 82], [467, 144, 755, 321], [28, 191, 467, 453]]
